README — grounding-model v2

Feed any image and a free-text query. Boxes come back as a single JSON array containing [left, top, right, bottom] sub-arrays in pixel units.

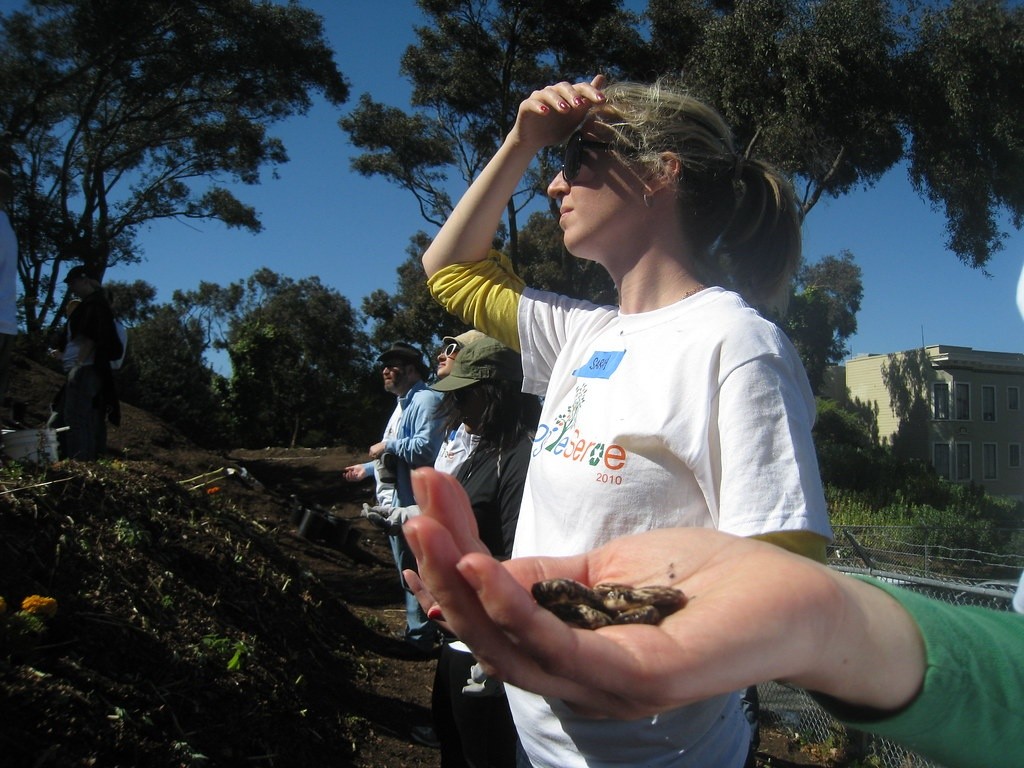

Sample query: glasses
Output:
[[562, 126, 645, 182], [379, 357, 410, 368], [444, 343, 461, 357], [450, 390, 472, 402]]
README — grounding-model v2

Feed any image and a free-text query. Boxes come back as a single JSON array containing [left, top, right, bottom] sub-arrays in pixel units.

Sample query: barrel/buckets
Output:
[[2, 427, 60, 465], [288, 495, 351, 549]]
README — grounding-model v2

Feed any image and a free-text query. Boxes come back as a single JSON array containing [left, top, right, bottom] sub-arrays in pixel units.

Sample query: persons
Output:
[[402, 256, 1024, 768], [421, 73, 832, 768], [0, 171, 128, 462], [342, 329, 545, 768]]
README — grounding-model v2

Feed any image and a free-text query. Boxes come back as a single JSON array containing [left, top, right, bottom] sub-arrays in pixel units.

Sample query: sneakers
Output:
[[409, 726, 441, 747]]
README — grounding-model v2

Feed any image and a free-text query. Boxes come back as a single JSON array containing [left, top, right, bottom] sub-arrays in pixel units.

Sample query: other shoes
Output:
[[385, 644, 430, 661]]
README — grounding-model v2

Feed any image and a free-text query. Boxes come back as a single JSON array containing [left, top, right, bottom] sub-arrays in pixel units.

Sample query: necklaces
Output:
[[682, 282, 706, 300]]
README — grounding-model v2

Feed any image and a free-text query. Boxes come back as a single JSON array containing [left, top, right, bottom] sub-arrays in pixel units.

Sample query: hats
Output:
[[377, 342, 422, 361], [443, 329, 487, 348], [428, 335, 523, 392]]
